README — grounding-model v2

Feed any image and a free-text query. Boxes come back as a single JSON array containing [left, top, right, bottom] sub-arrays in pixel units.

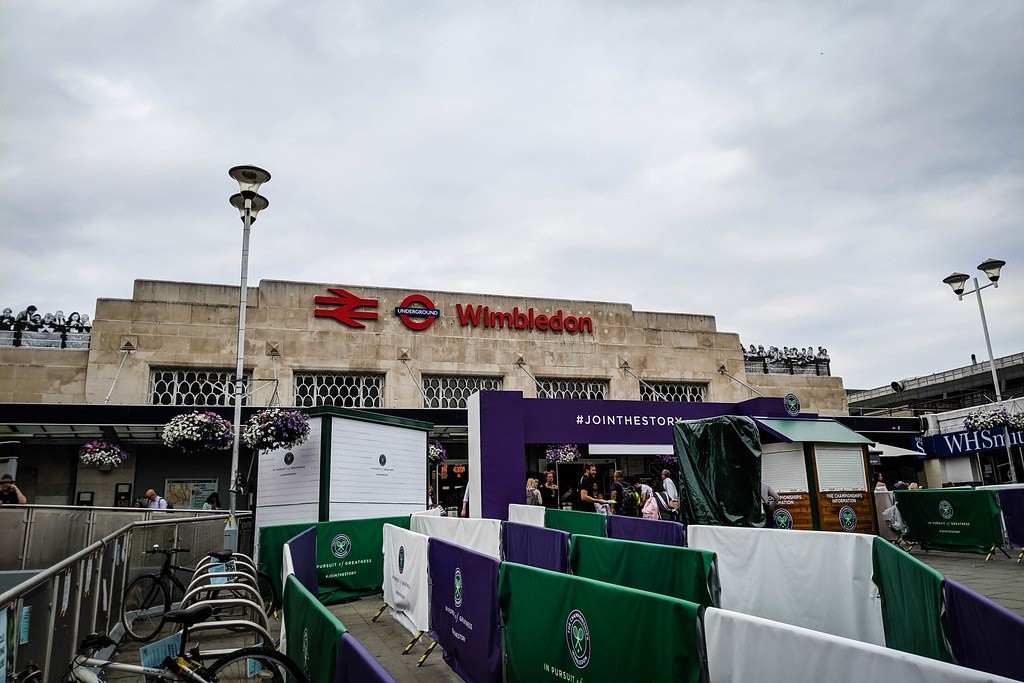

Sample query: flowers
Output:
[[964, 406, 1024, 433], [427, 439, 448, 466], [544, 442, 582, 464], [160, 408, 235, 454], [242, 407, 313, 455], [77, 436, 131, 471]]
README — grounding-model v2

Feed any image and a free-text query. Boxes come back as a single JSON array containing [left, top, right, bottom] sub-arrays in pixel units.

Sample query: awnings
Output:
[[868, 439, 928, 458], [753, 416, 873, 444]]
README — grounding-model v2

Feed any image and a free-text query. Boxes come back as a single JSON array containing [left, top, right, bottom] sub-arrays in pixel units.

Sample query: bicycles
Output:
[[60, 605, 309, 683], [121, 538, 277, 643]]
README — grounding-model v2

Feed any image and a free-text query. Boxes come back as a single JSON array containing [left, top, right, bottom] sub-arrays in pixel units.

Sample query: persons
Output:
[[571, 461, 680, 522], [0, 473, 27, 506], [760, 480, 781, 527], [539, 470, 559, 510], [461, 481, 469, 518], [428, 485, 437, 510], [526, 478, 544, 506], [0, 305, 91, 349], [873, 471, 888, 492], [131, 501, 144, 508], [739, 343, 831, 377], [202, 492, 222, 514], [145, 489, 168, 513]]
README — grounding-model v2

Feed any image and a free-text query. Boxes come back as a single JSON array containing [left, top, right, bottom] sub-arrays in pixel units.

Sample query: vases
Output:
[[274, 427, 293, 443], [429, 457, 441, 466]]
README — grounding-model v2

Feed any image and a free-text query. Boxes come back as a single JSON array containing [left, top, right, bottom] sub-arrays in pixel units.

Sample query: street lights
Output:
[[227, 163, 272, 517], [942, 258, 1018, 484]]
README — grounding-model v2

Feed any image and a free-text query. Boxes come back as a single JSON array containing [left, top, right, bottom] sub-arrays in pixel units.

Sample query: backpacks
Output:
[[617, 482, 638, 514], [526, 489, 539, 506], [641, 492, 661, 520]]
